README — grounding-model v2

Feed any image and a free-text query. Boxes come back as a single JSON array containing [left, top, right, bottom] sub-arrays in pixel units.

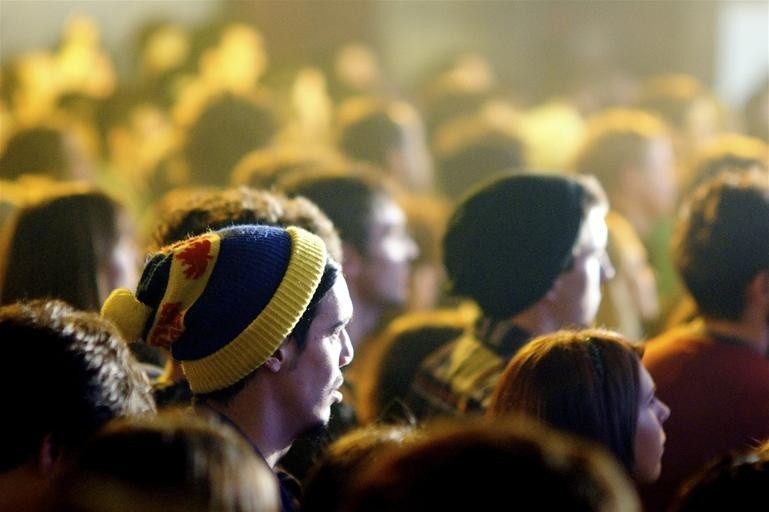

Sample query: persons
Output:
[[0, 19, 766, 508]]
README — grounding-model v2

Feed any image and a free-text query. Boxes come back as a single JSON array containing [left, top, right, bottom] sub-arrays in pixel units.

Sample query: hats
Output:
[[98, 225, 329, 397], [442, 176, 581, 318]]
